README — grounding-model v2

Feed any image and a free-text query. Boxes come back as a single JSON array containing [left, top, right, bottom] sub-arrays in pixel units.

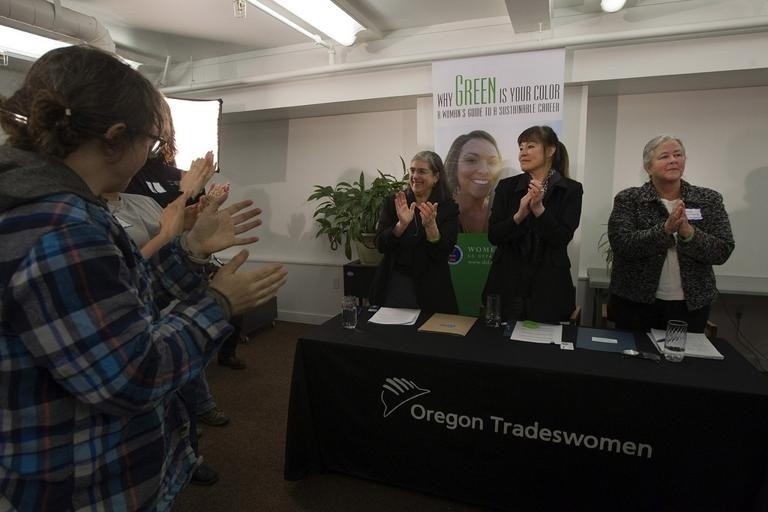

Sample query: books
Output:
[[647, 327, 724, 360], [417, 310, 479, 337]]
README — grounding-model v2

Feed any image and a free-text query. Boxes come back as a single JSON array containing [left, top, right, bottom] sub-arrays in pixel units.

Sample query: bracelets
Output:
[[676, 229, 695, 245]]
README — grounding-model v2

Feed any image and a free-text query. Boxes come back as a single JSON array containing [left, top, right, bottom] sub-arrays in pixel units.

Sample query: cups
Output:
[[663, 318, 688, 363], [339, 294, 360, 328], [486, 293, 502, 329]]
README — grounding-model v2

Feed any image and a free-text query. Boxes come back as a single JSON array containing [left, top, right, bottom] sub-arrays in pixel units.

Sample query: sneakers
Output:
[[196, 406, 231, 426]]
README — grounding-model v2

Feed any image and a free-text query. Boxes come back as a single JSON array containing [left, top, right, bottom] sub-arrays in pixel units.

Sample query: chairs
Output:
[[479, 284, 582, 325], [600, 298, 717, 338]]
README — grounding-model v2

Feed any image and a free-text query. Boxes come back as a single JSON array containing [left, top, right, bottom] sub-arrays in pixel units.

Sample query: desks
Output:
[[285, 308, 767, 511], [587, 266, 768, 328]]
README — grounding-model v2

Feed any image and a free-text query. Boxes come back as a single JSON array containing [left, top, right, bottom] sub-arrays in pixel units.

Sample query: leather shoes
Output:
[[216, 350, 247, 370], [190, 463, 220, 486]]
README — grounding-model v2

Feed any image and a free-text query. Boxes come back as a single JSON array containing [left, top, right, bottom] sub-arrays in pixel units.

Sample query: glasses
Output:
[[131, 129, 169, 157]]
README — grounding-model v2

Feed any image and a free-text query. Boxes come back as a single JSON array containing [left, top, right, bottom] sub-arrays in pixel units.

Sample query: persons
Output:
[[443, 130, 498, 230], [607, 134, 736, 336], [482, 126, 583, 324], [1, 43, 289, 511], [374, 151, 460, 315]]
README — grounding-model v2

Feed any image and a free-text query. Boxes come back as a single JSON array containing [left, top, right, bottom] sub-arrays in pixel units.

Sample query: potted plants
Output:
[[306, 155, 412, 265]]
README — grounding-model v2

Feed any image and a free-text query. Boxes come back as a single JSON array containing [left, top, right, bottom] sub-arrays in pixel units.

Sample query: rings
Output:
[[540, 188, 544, 192]]
